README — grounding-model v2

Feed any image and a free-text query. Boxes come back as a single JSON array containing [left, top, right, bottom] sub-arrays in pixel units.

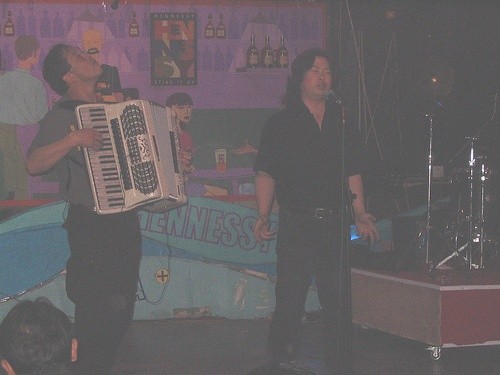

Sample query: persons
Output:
[[26, 43, 196, 375], [0, 297, 78, 375], [252, 48, 379, 375]]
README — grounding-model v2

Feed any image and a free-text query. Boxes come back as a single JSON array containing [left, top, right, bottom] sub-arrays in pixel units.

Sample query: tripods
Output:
[[411, 112, 500, 275]]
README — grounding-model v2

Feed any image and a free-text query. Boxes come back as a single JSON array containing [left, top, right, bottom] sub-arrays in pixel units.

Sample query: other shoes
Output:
[[269, 354, 314, 372]]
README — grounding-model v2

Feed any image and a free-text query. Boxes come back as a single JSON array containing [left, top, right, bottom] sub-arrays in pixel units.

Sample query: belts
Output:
[[290, 208, 343, 221]]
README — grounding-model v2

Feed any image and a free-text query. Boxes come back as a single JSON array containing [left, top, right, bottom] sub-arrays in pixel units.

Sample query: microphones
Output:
[[330, 90, 342, 105]]
